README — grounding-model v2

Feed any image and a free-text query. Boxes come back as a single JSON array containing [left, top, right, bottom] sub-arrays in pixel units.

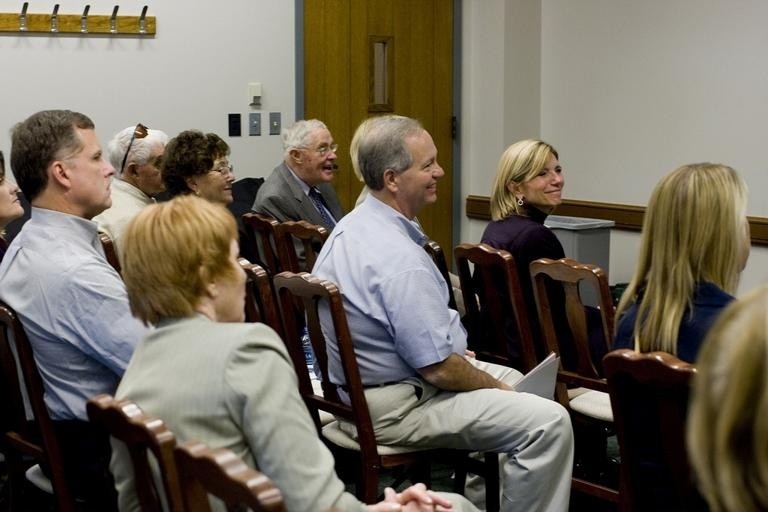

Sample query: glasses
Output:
[[210, 165, 234, 175], [297, 143, 339, 157], [120, 123, 149, 174]]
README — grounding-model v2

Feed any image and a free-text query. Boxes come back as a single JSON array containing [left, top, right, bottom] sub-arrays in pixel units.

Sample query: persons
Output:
[[307, 114, 574, 511], [159, 130, 233, 206], [684, 286, 766, 510], [110, 195, 480, 510], [92, 126, 167, 269], [2, 151, 24, 260], [251, 118, 340, 379], [477, 138, 564, 371], [1, 110, 153, 512], [609, 165, 750, 363]]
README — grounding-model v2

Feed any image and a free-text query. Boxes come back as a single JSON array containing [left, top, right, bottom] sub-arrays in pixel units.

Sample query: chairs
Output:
[[603, 348, 711, 512], [455, 242, 538, 375], [96, 230, 120, 274], [529, 256, 624, 512], [237, 256, 283, 340], [272, 270, 500, 512], [423, 239, 458, 310], [242, 211, 281, 276], [176, 438, 286, 512], [84, 391, 176, 512], [0, 301, 80, 512], [280, 220, 327, 275]]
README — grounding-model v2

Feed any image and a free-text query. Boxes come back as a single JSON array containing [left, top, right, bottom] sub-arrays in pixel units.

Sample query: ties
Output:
[[310, 188, 335, 228]]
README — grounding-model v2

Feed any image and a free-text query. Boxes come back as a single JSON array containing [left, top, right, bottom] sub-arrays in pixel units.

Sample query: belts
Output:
[[333, 378, 409, 392]]
